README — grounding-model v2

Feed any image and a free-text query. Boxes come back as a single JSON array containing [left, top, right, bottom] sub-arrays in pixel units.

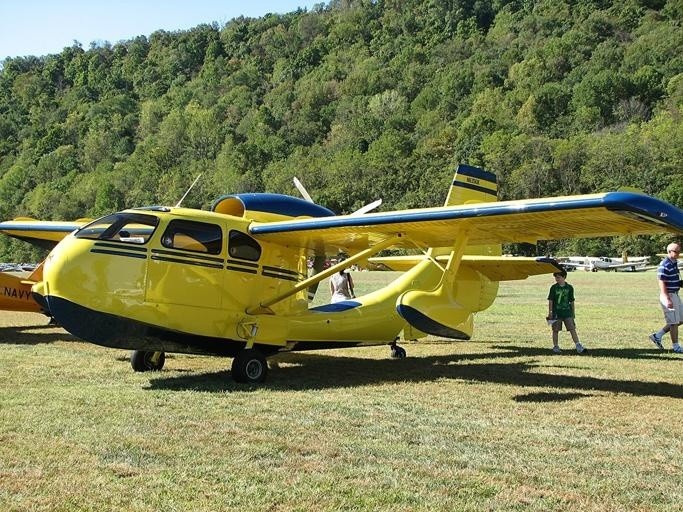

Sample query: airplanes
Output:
[[587, 253, 650, 272], [0, 160, 683, 386]]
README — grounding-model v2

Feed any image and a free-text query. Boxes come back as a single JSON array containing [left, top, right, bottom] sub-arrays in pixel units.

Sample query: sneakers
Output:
[[554, 345, 560, 353], [576, 344, 587, 354], [672, 345, 683, 352], [649, 333, 664, 351]]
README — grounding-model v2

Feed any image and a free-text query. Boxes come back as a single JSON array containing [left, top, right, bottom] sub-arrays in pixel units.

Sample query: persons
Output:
[[649, 242, 683, 353], [330, 257, 354, 305], [546, 270, 584, 354], [307, 256, 314, 275]]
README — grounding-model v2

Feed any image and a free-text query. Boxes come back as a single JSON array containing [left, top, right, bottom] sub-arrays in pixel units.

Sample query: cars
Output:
[[0, 261, 36, 272], [554, 256, 578, 271]]
